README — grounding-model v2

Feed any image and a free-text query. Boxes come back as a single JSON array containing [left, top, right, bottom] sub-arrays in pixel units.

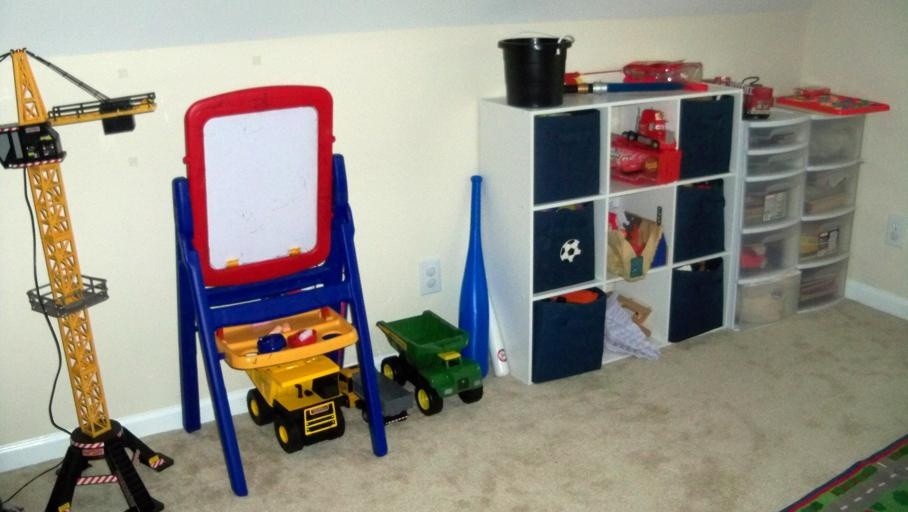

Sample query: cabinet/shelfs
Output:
[[473, 79, 746, 387]]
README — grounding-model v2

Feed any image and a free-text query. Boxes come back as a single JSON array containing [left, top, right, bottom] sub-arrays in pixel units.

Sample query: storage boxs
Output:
[[730, 100, 868, 333]]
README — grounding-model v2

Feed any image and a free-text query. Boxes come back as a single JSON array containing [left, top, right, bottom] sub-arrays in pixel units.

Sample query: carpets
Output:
[[776, 429, 908, 511]]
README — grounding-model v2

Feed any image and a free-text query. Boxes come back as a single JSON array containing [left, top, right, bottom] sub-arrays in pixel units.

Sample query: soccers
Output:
[[559, 239, 580, 262]]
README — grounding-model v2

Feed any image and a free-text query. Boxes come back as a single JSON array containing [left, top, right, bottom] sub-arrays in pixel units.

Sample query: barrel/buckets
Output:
[[497, 34, 576, 106]]
